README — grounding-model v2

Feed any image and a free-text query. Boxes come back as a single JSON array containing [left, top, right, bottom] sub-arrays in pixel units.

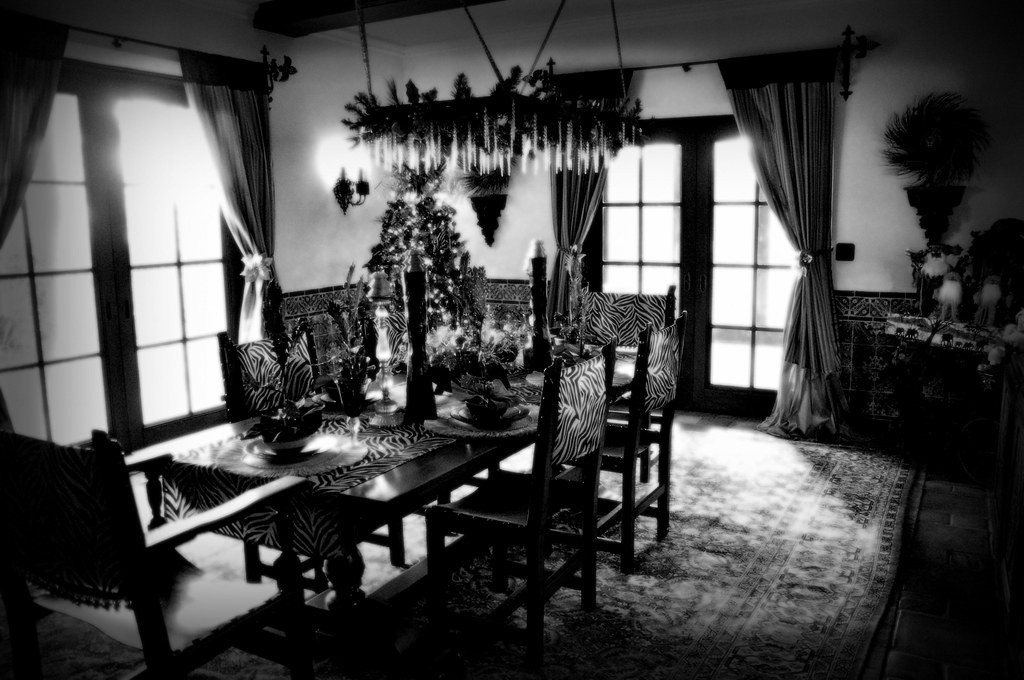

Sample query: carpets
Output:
[[0, 406, 930, 679]]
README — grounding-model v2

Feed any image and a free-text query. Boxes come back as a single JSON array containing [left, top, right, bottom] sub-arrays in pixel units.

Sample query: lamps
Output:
[[340, 0, 643, 175], [366, 266, 403, 429], [333, 164, 370, 214]]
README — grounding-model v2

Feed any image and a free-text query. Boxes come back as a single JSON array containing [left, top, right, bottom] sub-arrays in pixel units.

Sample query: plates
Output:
[[243, 439, 324, 460], [311, 392, 374, 407], [450, 405, 530, 426]]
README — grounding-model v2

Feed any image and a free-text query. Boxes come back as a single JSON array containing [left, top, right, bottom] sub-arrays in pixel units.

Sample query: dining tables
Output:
[[156, 344, 635, 680]]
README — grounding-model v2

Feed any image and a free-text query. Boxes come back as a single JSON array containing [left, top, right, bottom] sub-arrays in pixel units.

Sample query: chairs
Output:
[[428, 336, 618, 669], [0, 428, 313, 680], [215, 314, 406, 595], [562, 281, 677, 483], [550, 308, 689, 576]]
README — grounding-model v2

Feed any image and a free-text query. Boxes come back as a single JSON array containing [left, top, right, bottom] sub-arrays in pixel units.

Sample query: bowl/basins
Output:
[[465, 397, 508, 417], [324, 380, 369, 400], [264, 430, 318, 453]]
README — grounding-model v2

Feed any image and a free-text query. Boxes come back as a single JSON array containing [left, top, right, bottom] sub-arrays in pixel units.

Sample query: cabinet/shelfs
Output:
[[992, 351, 1024, 680]]
